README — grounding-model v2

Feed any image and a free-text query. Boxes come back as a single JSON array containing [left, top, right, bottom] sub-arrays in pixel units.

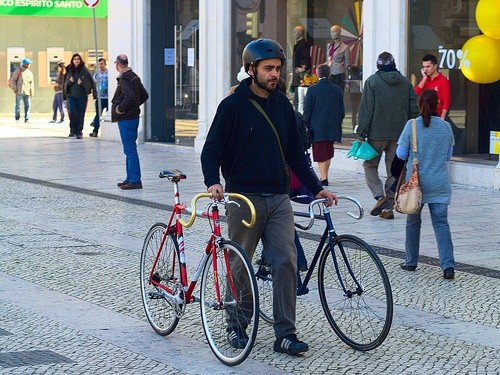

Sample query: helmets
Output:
[[242, 39, 287, 72]]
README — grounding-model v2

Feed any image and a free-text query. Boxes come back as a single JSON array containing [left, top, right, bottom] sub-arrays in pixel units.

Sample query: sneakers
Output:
[[274, 334, 308, 353], [226, 327, 249, 348]]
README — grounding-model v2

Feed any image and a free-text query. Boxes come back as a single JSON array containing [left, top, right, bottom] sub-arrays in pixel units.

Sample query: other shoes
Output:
[[380, 210, 394, 219], [401, 262, 416, 270], [118, 181, 126, 185], [120, 183, 142, 189], [90, 133, 97, 137], [69, 132, 74, 137], [321, 179, 328, 186], [444, 267, 454, 279], [77, 134, 82, 139], [370, 198, 389, 216]]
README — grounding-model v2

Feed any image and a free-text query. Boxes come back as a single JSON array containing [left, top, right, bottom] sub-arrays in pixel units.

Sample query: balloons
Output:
[[458, 0, 500, 84]]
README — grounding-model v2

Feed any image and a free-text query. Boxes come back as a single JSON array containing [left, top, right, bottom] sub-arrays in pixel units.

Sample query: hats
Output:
[[376, 52, 396, 69], [23, 58, 30, 64]]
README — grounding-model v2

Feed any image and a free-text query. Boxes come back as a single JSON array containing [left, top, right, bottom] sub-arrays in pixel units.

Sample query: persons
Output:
[[327, 25, 350, 91], [111, 54, 149, 190], [50, 62, 67, 123], [355, 52, 420, 219], [391, 89, 457, 279], [200, 40, 338, 355], [290, 26, 312, 110], [413, 55, 452, 121], [304, 64, 346, 186], [88, 59, 108, 138], [9, 57, 34, 123], [62, 53, 94, 139]]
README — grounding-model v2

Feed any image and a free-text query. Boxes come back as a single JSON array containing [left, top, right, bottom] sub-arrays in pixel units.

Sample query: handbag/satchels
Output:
[[289, 150, 322, 204], [395, 163, 422, 214], [285, 164, 301, 197], [306, 124, 313, 144], [346, 140, 380, 161]]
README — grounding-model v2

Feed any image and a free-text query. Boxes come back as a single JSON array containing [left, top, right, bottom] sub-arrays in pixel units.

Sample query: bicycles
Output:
[[247, 195, 394, 351], [139, 168, 261, 366]]
